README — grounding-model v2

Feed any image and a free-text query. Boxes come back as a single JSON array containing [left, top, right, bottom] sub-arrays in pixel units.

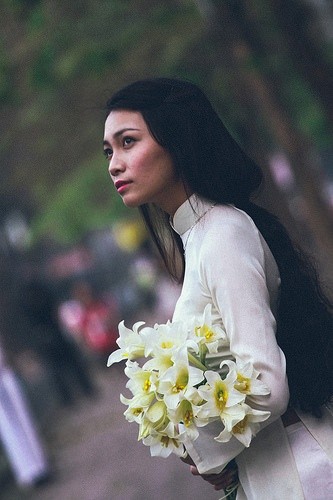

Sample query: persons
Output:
[[102, 77, 333, 500], [10, 267, 97, 410]]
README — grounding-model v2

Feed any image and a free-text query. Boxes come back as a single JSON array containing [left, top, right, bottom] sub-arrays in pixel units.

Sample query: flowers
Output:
[[108, 311, 268, 456]]
